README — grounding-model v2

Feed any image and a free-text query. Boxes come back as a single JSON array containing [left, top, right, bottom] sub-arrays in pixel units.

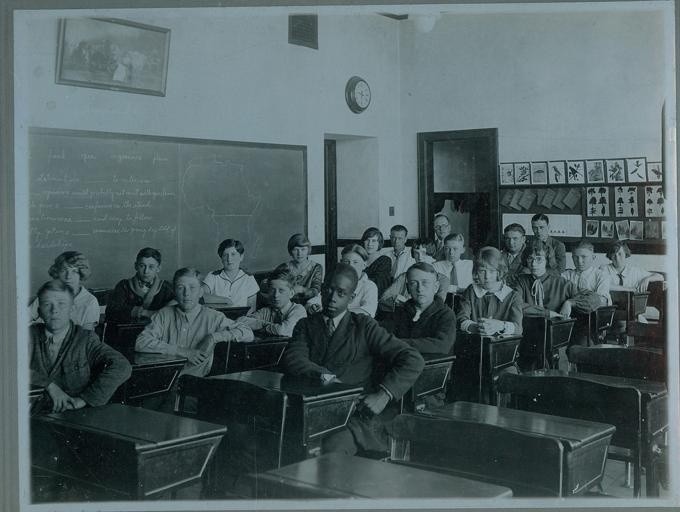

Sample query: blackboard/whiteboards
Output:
[[28, 127, 308, 305]]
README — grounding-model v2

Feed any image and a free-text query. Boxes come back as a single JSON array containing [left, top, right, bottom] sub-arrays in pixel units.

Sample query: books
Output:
[[498, 188, 582, 213]]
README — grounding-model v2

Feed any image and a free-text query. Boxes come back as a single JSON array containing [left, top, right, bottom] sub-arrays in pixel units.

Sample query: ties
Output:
[[617, 273, 625, 287], [449, 261, 458, 286], [274, 309, 283, 326], [391, 252, 404, 277], [326, 318, 335, 338]]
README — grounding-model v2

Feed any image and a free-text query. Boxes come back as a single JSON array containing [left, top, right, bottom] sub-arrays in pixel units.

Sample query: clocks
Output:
[[345, 76, 371, 114]]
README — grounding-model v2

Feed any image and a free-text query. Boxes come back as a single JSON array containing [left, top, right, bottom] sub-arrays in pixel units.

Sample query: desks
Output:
[[28, 281, 668, 503]]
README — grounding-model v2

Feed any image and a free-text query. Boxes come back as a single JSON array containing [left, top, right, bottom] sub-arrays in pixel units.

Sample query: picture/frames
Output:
[[55, 15, 171, 97]]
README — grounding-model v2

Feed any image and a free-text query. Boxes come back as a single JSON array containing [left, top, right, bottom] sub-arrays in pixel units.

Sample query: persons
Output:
[[234, 270, 307, 339], [105, 248, 175, 321], [29, 250, 133, 415], [560, 240, 611, 306], [598, 241, 664, 335], [135, 267, 254, 414], [262, 214, 567, 426], [511, 239, 602, 320], [200, 239, 261, 317]]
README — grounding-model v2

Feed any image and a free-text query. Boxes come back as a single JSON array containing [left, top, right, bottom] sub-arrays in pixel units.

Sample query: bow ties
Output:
[[530, 278, 544, 308]]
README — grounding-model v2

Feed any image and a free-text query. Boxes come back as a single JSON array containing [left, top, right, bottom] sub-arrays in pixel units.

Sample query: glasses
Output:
[[527, 255, 546, 265], [433, 224, 449, 230]]
